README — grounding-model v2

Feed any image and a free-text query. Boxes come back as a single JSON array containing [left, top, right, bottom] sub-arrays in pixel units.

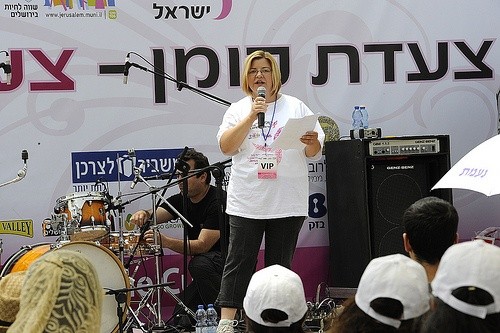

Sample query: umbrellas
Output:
[[430, 134, 500, 197]]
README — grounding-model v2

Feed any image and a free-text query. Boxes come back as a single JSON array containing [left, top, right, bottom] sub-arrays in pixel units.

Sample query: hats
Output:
[[242, 264, 307, 327], [355, 254, 431, 327], [0, 271, 28, 331], [431, 238, 500, 319]]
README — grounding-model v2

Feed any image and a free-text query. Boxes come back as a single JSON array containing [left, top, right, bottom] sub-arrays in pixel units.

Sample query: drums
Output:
[[0, 191, 153, 333]]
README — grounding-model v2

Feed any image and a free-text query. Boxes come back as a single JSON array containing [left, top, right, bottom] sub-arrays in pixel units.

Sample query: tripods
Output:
[[123, 176, 200, 333]]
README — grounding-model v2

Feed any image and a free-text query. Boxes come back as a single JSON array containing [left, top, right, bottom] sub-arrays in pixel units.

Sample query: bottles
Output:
[[359, 107, 369, 130], [195, 305, 207, 333], [352, 106, 363, 139], [205, 304, 218, 333]]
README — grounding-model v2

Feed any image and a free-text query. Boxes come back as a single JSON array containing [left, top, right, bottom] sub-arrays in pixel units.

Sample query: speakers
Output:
[[325, 135, 454, 289]]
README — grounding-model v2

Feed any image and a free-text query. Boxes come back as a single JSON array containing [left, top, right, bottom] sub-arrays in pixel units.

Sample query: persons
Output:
[[213, 51, 325, 333], [419, 227, 500, 333], [401, 197, 459, 333], [130, 148, 230, 331], [324, 253, 430, 333], [243, 264, 309, 333], [0, 250, 103, 333]]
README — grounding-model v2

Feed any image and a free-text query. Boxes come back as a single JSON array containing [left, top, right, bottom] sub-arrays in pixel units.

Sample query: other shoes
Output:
[[217, 319, 238, 333]]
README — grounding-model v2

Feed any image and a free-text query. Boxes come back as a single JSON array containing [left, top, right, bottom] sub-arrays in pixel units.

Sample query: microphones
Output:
[[257, 86, 266, 129], [124, 54, 130, 84], [94, 177, 102, 187], [130, 164, 144, 189]]
